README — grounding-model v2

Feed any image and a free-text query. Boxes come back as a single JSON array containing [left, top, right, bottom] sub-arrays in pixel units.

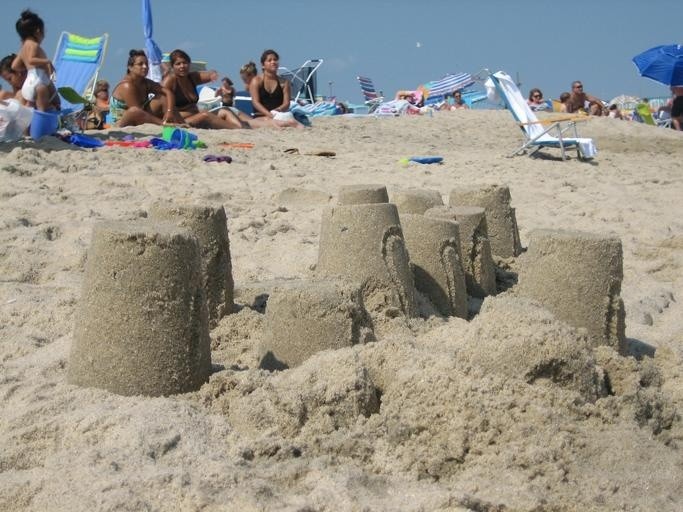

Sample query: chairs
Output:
[[546, 96, 565, 113], [37, 28, 108, 137], [633, 102, 675, 131], [355, 72, 412, 122], [479, 60, 597, 163]]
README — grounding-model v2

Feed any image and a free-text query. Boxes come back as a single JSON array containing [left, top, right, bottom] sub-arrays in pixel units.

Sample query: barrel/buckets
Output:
[[171, 127, 199, 150], [29, 109, 58, 142]]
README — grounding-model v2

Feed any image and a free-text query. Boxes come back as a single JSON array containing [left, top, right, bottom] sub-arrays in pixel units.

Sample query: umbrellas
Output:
[[140, 0, 160, 65], [421, 73, 476, 98], [631, 43, 683, 88]]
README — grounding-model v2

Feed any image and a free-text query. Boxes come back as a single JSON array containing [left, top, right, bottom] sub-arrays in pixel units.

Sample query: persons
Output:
[[394, 89, 470, 110], [527, 81, 622, 118], [670, 88, 683, 130], [94, 49, 304, 129], [1, 8, 60, 112]]
